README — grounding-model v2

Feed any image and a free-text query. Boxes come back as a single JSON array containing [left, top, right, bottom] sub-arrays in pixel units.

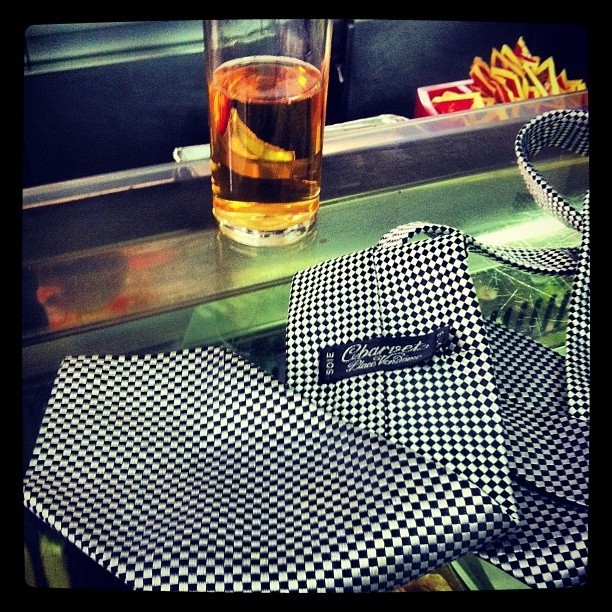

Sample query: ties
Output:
[[21, 108, 589, 593]]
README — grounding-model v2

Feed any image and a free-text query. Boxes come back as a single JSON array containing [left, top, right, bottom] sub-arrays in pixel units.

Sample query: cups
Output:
[[202, 18, 335, 248]]
